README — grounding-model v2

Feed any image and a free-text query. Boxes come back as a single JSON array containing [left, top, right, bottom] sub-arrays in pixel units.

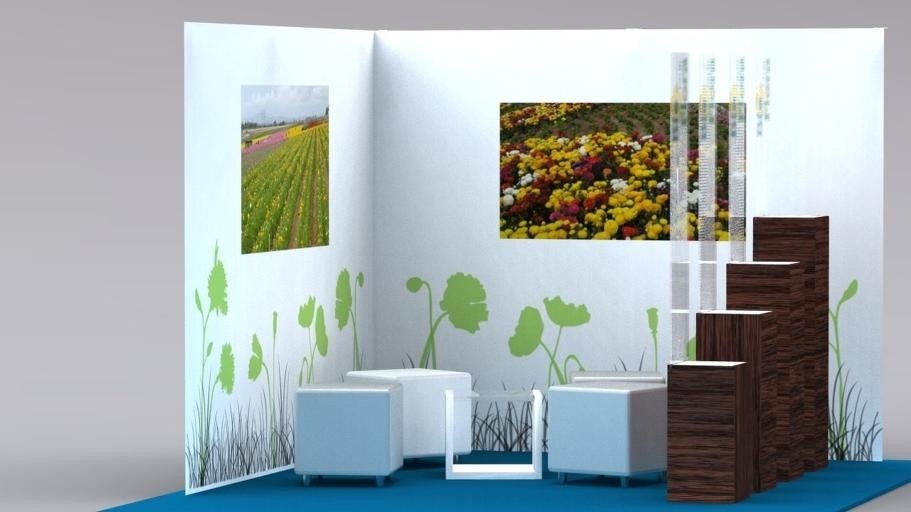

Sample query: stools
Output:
[[295, 368, 472, 486], [549, 371, 668, 487]]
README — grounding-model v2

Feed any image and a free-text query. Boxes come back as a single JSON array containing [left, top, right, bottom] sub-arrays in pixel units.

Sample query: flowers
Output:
[[500, 102, 730, 241]]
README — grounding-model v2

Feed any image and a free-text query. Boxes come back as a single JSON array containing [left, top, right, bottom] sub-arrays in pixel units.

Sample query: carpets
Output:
[[94, 450, 911, 511]]
